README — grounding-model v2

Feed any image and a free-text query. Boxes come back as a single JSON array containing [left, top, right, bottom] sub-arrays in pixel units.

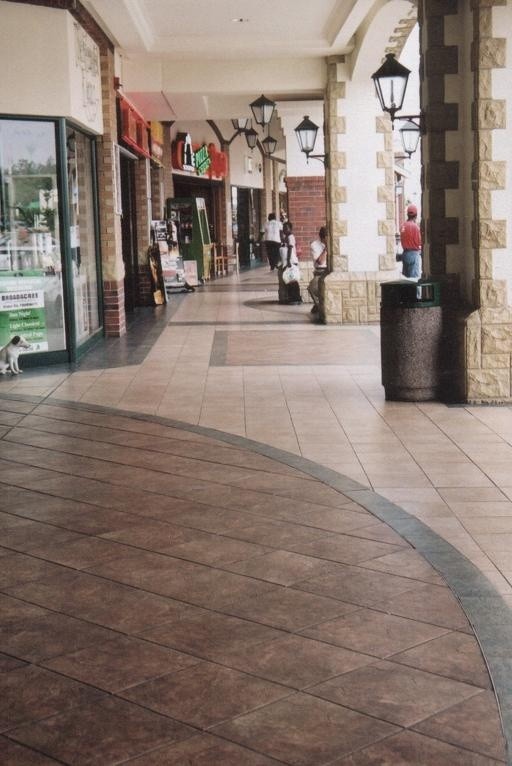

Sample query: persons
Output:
[[397, 204, 423, 280], [273, 222, 303, 304], [259, 212, 285, 270], [306, 225, 329, 313]]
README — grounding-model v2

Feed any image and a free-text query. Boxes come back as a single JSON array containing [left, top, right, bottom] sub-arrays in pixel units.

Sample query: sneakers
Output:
[[271, 264, 278, 270]]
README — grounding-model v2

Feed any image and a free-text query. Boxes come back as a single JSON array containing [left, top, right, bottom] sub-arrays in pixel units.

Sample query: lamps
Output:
[[293, 115, 326, 166], [230, 93, 278, 159], [370, 51, 426, 161]]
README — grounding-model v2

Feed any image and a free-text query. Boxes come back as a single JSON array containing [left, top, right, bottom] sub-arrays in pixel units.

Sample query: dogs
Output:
[[1, 334, 32, 376]]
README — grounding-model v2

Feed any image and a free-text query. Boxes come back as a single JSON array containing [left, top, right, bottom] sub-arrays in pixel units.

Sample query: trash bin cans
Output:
[[380, 279, 442, 403]]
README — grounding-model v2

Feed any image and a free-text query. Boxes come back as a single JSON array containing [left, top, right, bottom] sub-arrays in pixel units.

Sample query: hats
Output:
[[408, 205, 417, 216]]
